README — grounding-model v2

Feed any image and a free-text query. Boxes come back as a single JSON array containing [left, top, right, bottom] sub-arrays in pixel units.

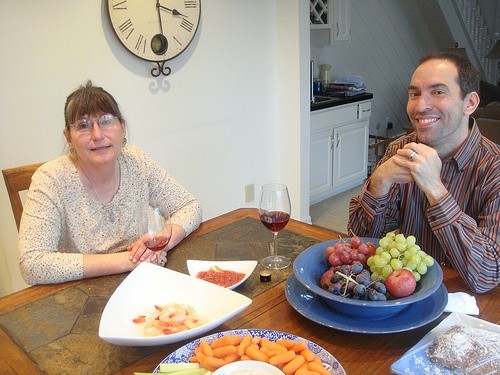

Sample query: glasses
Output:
[[68, 114, 119, 132]]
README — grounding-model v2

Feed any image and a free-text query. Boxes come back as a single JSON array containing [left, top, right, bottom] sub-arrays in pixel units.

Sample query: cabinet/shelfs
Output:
[[309, 98, 373, 206], [310, 0, 353, 46]]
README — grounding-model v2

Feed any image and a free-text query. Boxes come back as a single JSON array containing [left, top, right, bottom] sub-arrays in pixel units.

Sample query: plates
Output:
[[187, 259, 258, 290], [98, 261, 253, 346], [390, 311, 500, 375], [284, 273, 448, 334], [152, 328, 347, 375]]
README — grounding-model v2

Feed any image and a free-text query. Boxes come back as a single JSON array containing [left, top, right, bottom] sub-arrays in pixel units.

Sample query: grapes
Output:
[[326, 228, 434, 300]]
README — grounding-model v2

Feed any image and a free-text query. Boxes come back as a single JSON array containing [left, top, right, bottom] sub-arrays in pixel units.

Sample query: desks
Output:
[[0, 208, 500, 375]]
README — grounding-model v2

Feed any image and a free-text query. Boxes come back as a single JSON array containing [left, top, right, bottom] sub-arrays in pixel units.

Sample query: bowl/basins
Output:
[[293, 237, 443, 319]]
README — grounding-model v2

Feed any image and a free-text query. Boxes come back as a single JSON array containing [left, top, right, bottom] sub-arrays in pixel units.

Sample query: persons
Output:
[[17, 81, 201, 285], [347, 51, 500, 293]]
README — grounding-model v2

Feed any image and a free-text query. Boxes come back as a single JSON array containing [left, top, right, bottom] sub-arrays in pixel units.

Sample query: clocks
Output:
[[107, 1, 201, 77]]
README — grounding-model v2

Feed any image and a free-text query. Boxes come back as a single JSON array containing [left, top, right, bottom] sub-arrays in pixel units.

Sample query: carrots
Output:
[[190, 335, 333, 375]]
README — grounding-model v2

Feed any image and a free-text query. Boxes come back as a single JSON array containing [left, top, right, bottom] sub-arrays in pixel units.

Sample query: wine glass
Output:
[[259, 183, 292, 270], [138, 206, 172, 267]]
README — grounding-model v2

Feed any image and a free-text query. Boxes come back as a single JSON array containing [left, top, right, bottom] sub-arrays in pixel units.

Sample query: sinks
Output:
[[312, 95, 339, 105]]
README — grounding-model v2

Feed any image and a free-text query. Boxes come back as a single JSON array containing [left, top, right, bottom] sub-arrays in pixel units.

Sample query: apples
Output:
[[322, 269, 334, 288], [386, 269, 416, 297]]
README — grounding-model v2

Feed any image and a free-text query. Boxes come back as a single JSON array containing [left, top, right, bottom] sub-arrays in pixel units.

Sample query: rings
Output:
[[160, 259, 163, 264], [410, 152, 416, 161]]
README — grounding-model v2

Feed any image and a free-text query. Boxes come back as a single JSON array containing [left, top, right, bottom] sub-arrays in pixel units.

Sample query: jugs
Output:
[[319, 63, 331, 85]]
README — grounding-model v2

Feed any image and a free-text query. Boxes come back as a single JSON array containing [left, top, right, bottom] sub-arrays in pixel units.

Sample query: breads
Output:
[[428, 325, 500, 375]]
